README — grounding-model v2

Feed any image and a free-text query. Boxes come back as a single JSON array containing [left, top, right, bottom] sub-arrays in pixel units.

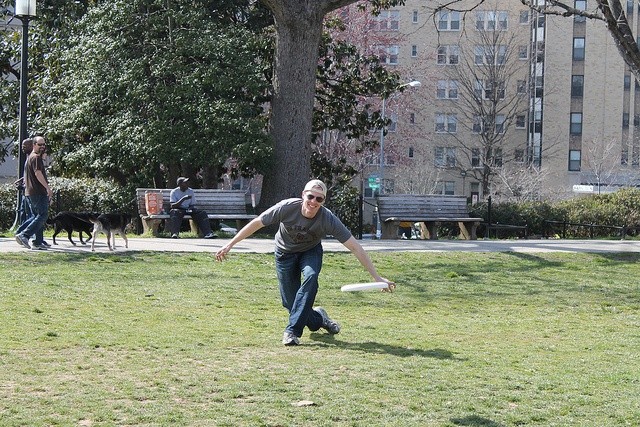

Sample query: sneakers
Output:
[[31, 242, 48, 250], [13, 230, 22, 245], [43, 241, 51, 248], [16, 232, 31, 249], [313, 306, 341, 334], [172, 233, 178, 239], [204, 232, 218, 238], [281, 332, 299, 347]]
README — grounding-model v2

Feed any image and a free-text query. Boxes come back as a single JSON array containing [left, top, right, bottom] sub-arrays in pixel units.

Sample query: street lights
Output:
[[14, 0, 37, 227], [377, 81, 421, 237]]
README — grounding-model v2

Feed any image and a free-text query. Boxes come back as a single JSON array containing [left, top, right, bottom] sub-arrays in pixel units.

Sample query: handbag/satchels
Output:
[[144, 189, 163, 216]]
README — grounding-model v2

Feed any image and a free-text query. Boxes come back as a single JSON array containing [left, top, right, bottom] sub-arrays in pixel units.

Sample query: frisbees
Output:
[[341, 281, 389, 292]]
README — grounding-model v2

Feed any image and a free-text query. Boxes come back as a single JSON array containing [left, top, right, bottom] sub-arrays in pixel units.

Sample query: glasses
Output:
[[44, 151, 48, 154], [34, 140, 46, 146], [304, 191, 325, 202]]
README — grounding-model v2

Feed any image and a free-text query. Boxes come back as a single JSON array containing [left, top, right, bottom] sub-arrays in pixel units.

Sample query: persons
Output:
[[13, 138, 35, 237], [15, 136, 53, 250], [168, 176, 218, 239], [14, 144, 52, 247], [215, 179, 397, 346]]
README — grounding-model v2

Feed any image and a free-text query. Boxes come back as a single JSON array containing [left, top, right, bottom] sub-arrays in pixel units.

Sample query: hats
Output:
[[177, 177, 189, 186], [304, 179, 328, 197]]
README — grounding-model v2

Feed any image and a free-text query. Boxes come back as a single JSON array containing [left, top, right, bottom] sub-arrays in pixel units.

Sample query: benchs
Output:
[[135, 187, 259, 246], [376, 193, 484, 238]]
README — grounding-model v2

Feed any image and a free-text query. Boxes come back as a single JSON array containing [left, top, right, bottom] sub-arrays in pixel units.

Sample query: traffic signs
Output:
[[368, 177, 380, 188]]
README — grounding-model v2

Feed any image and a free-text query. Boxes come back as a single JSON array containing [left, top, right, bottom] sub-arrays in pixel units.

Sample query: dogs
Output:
[[47, 213, 100, 246], [88, 212, 131, 251]]
